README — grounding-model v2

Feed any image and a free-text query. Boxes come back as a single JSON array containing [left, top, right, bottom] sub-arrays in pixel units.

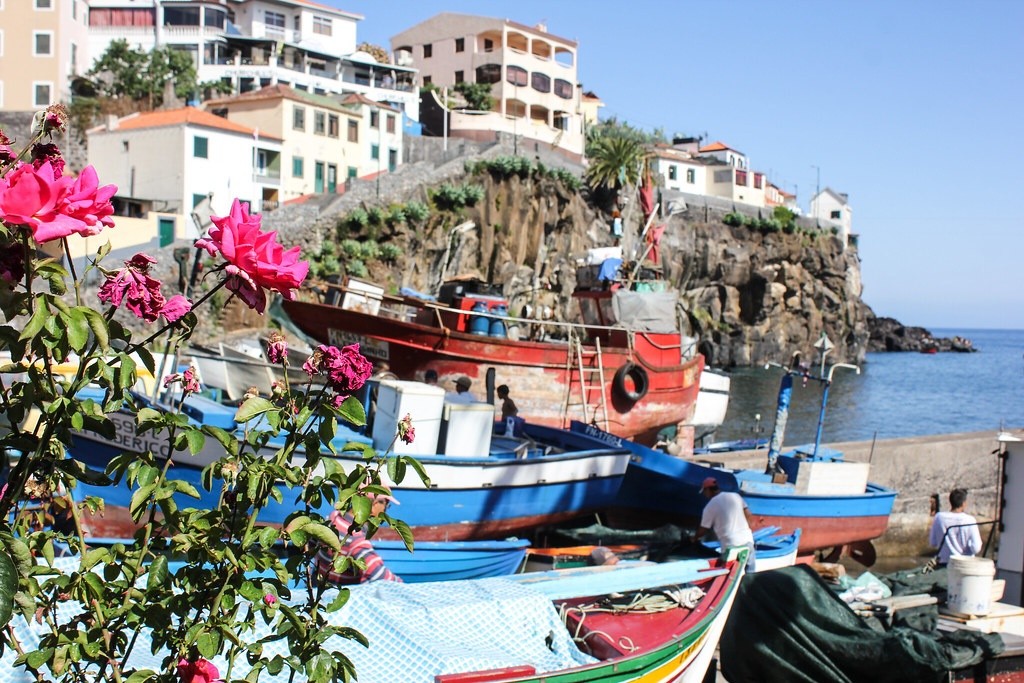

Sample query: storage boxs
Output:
[[332, 377, 379, 429], [371, 408, 440, 455], [440, 401, 495, 457], [378, 381, 445, 420]]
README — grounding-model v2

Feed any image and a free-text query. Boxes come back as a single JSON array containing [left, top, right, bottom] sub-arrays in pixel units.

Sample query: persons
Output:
[[696, 477, 755, 573], [425, 369, 439, 386], [930, 489, 983, 601], [498, 384, 519, 420], [451, 377, 476, 403], [375, 363, 395, 380]]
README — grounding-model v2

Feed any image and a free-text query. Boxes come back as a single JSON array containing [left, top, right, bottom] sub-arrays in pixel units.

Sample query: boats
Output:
[[299, 155, 734, 446], [729, 559, 1024, 682], [713, 336, 900, 568], [2, 320, 762, 683]]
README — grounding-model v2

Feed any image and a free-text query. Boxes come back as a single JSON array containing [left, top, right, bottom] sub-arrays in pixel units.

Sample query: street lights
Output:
[[808, 164, 821, 219]]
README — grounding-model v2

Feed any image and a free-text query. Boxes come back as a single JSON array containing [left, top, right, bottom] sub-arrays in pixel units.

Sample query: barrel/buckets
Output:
[[948, 555, 994, 615]]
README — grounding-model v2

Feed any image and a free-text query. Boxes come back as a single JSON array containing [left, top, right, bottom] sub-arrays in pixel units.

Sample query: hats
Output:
[[699, 477, 717, 493], [452, 377, 472, 387]]
